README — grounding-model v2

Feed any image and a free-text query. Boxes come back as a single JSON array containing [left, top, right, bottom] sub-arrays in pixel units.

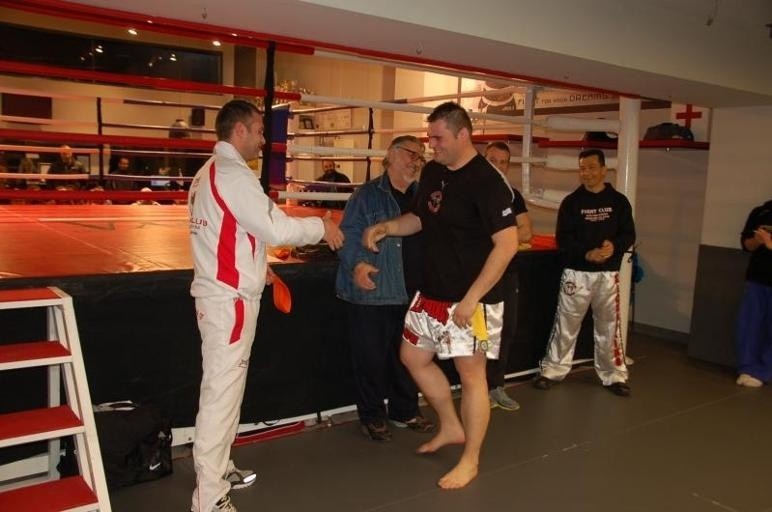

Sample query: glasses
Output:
[[394, 145, 427, 165]]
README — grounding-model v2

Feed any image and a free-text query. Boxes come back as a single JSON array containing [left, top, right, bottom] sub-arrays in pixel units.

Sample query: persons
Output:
[[482, 140, 531, 412], [186, 98, 345, 512], [535, 148, 637, 396], [361, 100, 519, 489], [300, 159, 355, 209], [335, 134, 436, 443], [736, 198, 772, 388], [1, 145, 188, 205]]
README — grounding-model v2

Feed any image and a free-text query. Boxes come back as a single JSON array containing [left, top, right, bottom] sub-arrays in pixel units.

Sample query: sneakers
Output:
[[604, 381, 631, 396], [391, 412, 439, 434], [487, 385, 520, 412], [360, 415, 392, 442], [191, 494, 239, 512], [735, 372, 764, 389], [533, 375, 559, 390], [222, 461, 258, 491]]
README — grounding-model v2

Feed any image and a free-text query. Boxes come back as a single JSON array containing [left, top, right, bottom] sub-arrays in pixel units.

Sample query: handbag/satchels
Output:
[[55, 400, 174, 494]]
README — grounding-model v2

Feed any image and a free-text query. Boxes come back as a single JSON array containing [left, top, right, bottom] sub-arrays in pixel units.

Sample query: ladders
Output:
[[0, 287, 113, 511]]
